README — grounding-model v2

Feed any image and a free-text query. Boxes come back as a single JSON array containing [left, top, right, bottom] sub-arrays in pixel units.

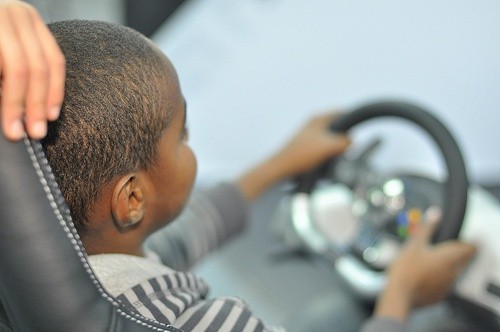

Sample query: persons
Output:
[[36, 17, 478, 332], [0, 0, 67, 142]]
